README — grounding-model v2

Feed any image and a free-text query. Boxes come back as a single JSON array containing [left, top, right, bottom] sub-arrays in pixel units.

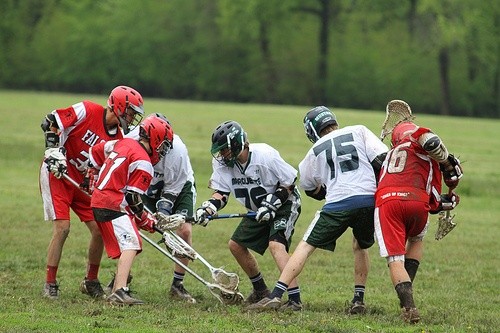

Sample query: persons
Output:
[[246, 106, 389, 315], [191, 120, 302, 312], [38, 86, 144, 301], [109, 126, 197, 304], [87, 114, 173, 305], [374, 122, 463, 322]]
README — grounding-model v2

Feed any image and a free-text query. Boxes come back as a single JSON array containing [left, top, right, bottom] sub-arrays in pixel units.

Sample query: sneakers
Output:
[[245, 290, 272, 304], [107, 287, 144, 305], [107, 271, 134, 290], [345, 300, 365, 315], [248, 297, 282, 310], [42, 282, 61, 300], [279, 299, 303, 312], [78, 277, 109, 302], [170, 284, 196, 304]]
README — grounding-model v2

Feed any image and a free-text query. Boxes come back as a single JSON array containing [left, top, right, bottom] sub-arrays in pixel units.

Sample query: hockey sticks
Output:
[[381, 98, 413, 142], [435, 185, 456, 241], [156, 211, 257, 231], [153, 225, 196, 260], [144, 204, 239, 292], [61, 172, 245, 304]]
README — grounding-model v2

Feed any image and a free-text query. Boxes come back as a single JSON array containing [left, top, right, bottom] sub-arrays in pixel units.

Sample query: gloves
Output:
[[79, 167, 93, 192], [44, 147, 69, 179], [441, 154, 463, 189], [441, 191, 460, 211], [156, 199, 174, 216], [135, 210, 156, 233], [192, 201, 217, 227], [255, 193, 283, 223]]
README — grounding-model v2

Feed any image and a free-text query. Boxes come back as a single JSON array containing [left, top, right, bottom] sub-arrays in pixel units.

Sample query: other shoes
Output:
[[401, 307, 420, 323]]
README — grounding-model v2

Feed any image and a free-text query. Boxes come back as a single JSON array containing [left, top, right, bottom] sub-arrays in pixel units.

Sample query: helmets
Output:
[[211, 120, 247, 168], [304, 105, 338, 144], [107, 86, 144, 135], [390, 121, 418, 148], [139, 113, 173, 166]]
